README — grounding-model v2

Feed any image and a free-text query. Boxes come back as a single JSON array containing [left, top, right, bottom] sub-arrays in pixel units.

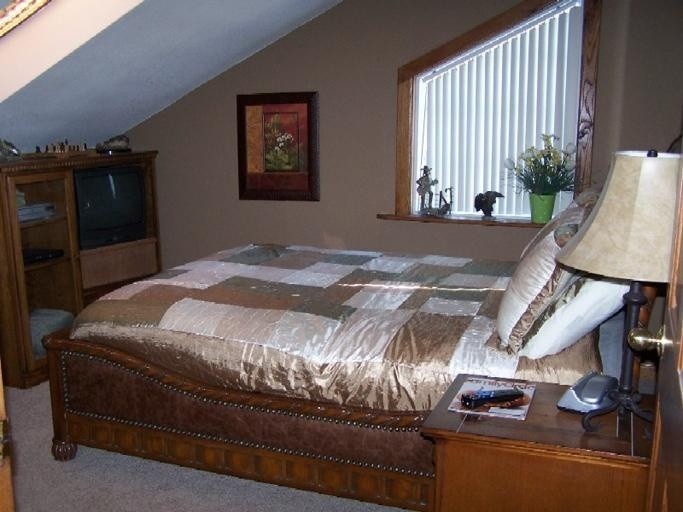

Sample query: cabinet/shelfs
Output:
[[0, 159, 80, 390]]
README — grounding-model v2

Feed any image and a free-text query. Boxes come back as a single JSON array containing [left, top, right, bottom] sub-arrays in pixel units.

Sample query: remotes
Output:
[[461, 389, 524, 410]]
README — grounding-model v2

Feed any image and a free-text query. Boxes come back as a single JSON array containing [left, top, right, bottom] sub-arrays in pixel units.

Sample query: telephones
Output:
[[557, 373, 619, 415]]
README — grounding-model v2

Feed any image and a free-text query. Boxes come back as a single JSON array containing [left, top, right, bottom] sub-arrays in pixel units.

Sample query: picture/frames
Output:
[[235, 91, 321, 201]]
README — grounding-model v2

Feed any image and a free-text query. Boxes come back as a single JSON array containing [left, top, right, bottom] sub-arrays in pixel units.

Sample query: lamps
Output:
[[552, 148, 680, 438]]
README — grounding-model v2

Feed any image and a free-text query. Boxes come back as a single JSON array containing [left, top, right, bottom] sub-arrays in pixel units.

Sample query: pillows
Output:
[[496, 185, 599, 357], [517, 277, 632, 359]]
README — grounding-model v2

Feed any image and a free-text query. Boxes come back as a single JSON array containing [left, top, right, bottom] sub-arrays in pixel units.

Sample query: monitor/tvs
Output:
[[74, 164, 147, 250]]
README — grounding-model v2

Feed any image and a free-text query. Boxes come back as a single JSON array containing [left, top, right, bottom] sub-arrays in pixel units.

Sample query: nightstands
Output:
[[420, 373, 654, 510]]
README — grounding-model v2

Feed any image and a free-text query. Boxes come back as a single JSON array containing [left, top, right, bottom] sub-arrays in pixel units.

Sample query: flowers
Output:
[[500, 133, 578, 195]]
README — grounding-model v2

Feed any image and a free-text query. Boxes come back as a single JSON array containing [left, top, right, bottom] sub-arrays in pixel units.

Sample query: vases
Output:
[[529, 191, 556, 224]]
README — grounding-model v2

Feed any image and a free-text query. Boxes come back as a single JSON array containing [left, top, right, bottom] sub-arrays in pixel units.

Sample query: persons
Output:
[[416, 166, 437, 209]]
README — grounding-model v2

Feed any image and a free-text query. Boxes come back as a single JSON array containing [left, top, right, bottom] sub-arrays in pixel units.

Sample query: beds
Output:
[[40, 128, 680, 510]]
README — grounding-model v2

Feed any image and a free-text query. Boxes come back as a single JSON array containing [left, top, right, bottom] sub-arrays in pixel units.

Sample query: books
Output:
[[448, 377, 537, 421]]
